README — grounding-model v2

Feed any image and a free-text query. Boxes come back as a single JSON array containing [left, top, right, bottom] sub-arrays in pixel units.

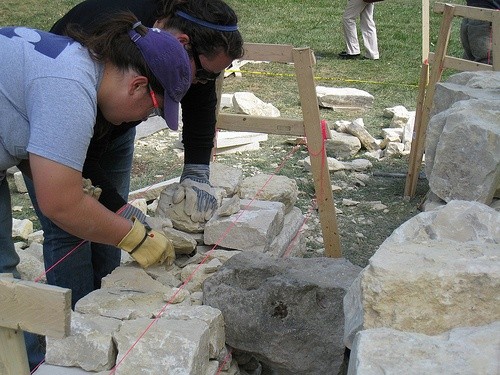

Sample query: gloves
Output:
[[172, 164, 218, 222], [116, 216, 175, 269], [83, 179, 101, 201], [119, 203, 172, 236]]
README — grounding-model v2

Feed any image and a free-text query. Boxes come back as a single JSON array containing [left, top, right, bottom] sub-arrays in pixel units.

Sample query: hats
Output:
[[128, 27, 191, 131]]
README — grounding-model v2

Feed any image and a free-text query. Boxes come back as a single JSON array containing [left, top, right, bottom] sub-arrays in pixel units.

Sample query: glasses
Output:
[[191, 48, 221, 81], [147, 84, 163, 117]]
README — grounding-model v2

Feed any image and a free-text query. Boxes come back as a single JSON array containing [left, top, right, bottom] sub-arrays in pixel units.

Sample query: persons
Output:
[[338, 0, 385, 60], [460, 0, 500, 65], [20, 0, 246, 311], [0, 10, 192, 373]]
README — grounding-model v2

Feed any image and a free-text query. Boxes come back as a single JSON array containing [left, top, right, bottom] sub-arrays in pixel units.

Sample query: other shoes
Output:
[[338, 51, 361, 57]]
